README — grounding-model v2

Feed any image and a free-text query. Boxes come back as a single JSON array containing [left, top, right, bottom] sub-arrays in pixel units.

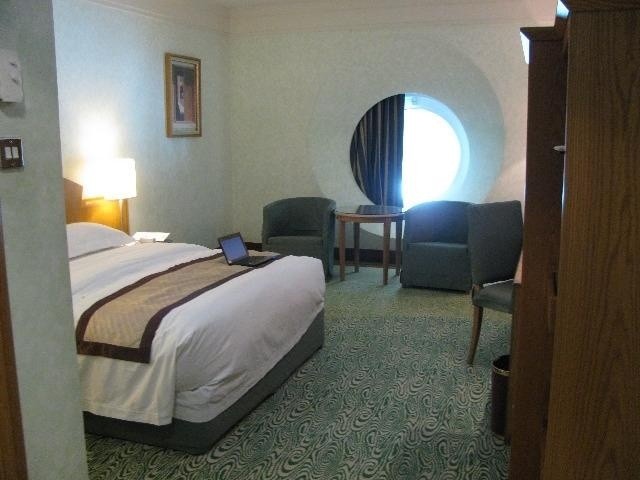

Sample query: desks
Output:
[[336, 206, 404, 284]]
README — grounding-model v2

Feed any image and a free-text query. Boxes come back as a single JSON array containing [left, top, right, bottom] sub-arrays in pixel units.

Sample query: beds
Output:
[[61, 178, 328, 455]]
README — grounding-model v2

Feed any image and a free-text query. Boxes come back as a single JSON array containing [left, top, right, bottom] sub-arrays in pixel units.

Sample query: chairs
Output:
[[464, 200, 524, 365]]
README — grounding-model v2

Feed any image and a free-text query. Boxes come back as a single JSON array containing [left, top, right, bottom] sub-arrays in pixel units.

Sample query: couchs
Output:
[[401, 201, 474, 293], [260, 197, 336, 282]]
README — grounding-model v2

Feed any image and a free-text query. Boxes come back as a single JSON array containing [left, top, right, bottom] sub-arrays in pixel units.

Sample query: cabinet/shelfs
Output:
[[510, 0, 640, 480]]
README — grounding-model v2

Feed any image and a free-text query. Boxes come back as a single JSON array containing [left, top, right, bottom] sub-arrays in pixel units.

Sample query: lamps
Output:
[[101, 160, 139, 232]]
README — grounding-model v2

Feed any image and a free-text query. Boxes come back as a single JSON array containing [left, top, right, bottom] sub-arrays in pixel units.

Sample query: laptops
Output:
[[217, 232, 273, 267]]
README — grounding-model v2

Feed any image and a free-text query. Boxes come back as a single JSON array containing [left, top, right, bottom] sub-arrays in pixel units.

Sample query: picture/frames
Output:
[[165, 51, 201, 139]]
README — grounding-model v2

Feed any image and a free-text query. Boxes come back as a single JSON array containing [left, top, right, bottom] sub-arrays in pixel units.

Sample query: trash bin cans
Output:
[[492, 355, 509, 434]]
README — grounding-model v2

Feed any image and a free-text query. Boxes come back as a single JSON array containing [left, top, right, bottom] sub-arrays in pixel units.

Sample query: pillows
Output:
[[65, 222, 133, 261]]
